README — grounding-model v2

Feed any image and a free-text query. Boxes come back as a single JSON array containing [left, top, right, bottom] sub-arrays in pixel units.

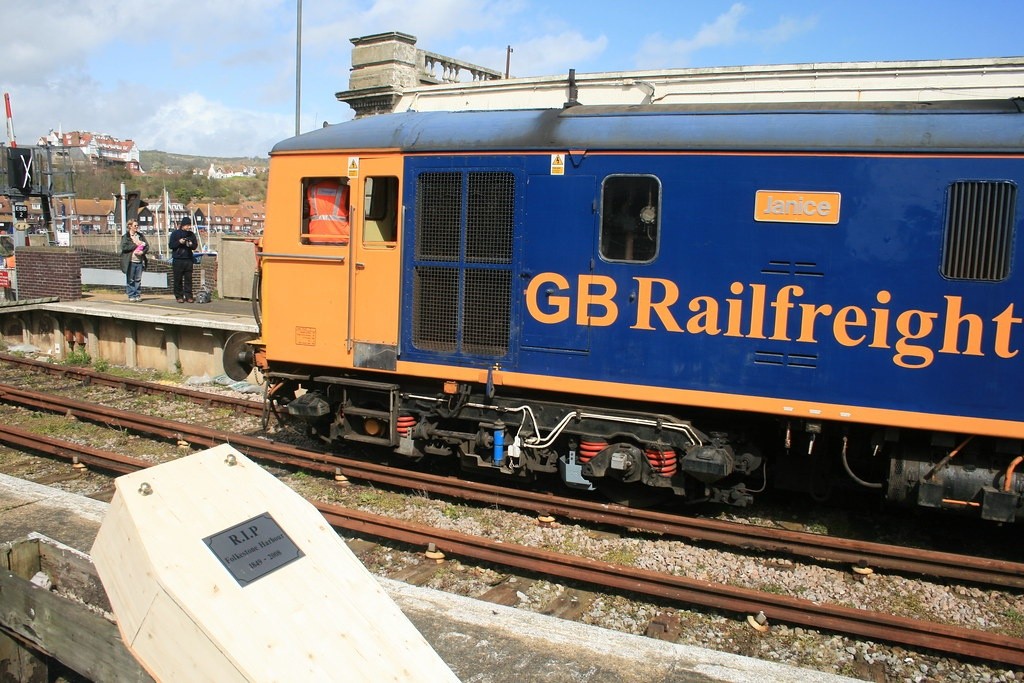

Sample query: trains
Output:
[[222, 95, 1024, 550]]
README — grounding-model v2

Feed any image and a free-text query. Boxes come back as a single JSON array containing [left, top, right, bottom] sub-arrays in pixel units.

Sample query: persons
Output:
[[168, 218, 197, 302], [120, 219, 149, 302], [303, 177, 350, 245]]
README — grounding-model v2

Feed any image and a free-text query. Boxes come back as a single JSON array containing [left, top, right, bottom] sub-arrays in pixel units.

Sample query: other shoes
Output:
[[186, 298, 194, 303], [176, 297, 184, 303], [136, 296, 142, 301], [129, 297, 136, 302]]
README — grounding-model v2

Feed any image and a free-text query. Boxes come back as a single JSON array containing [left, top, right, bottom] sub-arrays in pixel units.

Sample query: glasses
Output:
[[130, 224, 138, 227]]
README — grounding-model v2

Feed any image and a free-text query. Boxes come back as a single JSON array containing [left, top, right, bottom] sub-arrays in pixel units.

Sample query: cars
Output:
[[167, 252, 217, 264]]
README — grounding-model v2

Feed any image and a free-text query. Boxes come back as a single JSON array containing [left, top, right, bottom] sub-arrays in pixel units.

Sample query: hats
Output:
[[181, 217, 191, 226]]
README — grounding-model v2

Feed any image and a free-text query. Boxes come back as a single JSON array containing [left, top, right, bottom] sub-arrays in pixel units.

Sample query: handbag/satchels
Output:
[[196, 285, 211, 303]]
